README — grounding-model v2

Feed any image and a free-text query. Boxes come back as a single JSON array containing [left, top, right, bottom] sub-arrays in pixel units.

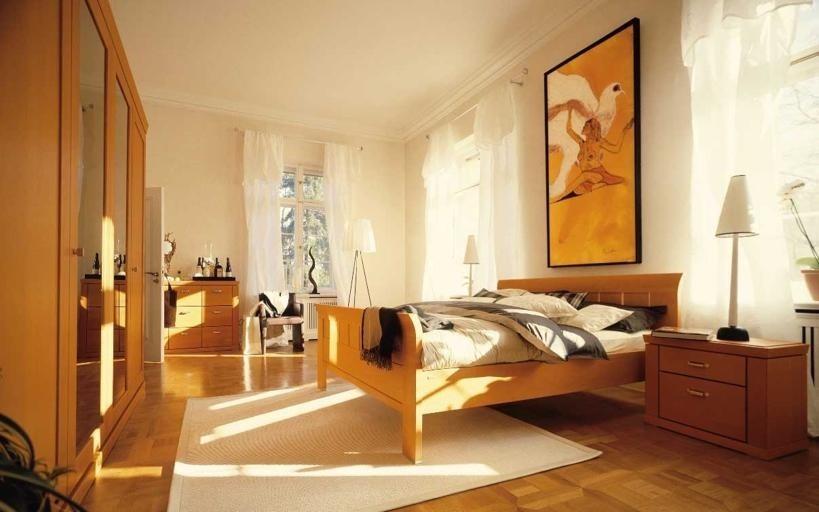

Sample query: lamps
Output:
[[345, 218, 377, 308], [464, 236, 479, 296], [715, 175, 760, 342]]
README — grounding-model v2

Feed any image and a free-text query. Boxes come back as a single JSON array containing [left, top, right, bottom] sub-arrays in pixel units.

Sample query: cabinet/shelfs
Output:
[[79, 279, 127, 363], [164, 280, 240, 355], [0, 0, 148, 512]]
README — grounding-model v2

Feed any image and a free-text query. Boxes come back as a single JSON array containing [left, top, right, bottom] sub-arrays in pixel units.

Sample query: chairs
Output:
[[258, 290, 305, 354]]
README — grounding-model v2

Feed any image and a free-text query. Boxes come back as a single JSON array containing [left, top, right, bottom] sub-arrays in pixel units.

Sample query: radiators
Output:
[[284, 294, 338, 342]]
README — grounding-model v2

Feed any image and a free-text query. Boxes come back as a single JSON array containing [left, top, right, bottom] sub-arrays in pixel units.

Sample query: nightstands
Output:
[[643, 330, 810, 461]]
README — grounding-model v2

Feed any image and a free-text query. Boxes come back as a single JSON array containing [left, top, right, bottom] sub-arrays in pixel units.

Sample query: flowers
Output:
[[777, 176, 819, 270]]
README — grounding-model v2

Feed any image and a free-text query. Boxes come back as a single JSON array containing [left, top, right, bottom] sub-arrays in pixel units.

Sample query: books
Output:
[[651, 326, 714, 341]]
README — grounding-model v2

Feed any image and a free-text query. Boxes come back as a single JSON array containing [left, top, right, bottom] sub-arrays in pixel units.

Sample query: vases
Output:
[[799, 270, 819, 302]]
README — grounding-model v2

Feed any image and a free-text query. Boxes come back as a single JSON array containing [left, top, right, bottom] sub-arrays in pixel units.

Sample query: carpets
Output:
[[166, 377, 602, 512]]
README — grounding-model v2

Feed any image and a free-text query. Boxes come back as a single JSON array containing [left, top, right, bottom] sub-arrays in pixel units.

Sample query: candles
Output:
[[117, 239, 120, 256], [210, 243, 212, 261]]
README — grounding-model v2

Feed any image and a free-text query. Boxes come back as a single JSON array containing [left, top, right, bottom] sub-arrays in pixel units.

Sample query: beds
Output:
[[315, 273, 684, 464]]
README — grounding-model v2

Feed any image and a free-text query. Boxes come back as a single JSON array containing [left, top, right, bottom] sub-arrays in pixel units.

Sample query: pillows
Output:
[[473, 288, 667, 335]]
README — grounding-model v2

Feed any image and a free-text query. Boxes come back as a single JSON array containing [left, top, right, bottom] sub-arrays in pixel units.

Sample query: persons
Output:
[[556, 107, 634, 205]]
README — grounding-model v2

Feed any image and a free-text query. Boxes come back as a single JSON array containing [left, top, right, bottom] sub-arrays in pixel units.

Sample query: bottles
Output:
[[92, 252, 100, 274], [195, 256, 232, 277]]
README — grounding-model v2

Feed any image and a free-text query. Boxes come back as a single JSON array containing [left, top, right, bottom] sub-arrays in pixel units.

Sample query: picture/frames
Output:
[[543, 17, 642, 268]]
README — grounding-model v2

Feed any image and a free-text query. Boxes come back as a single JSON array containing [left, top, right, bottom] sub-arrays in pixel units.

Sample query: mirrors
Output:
[[113, 75, 130, 405], [70, 0, 108, 458]]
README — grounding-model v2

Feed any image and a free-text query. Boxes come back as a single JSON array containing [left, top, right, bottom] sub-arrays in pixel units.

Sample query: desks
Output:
[[794, 303, 818, 439]]
